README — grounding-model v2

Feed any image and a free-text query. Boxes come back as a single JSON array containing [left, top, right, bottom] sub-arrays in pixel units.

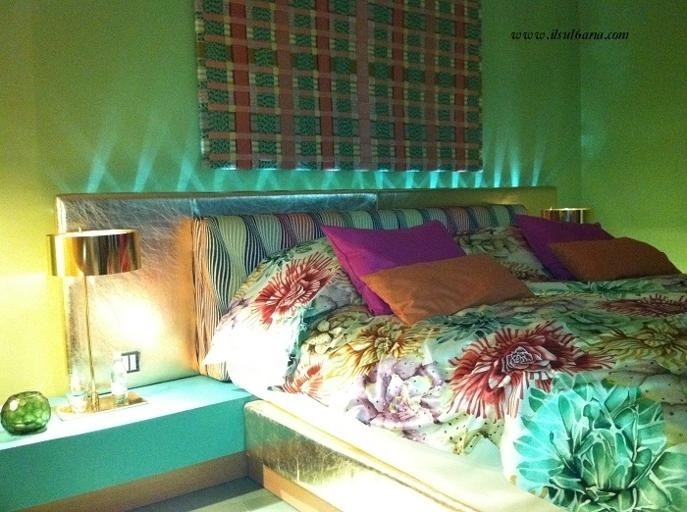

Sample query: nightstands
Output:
[[1, 372, 260, 511]]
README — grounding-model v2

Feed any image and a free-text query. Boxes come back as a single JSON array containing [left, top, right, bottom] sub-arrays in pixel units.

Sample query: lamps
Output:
[[48, 227, 147, 422], [56, 187, 686, 512], [543, 207, 593, 224]]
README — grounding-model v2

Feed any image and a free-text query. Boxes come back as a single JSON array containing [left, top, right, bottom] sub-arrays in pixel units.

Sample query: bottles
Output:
[[66, 362, 90, 415], [110, 353, 129, 406]]
